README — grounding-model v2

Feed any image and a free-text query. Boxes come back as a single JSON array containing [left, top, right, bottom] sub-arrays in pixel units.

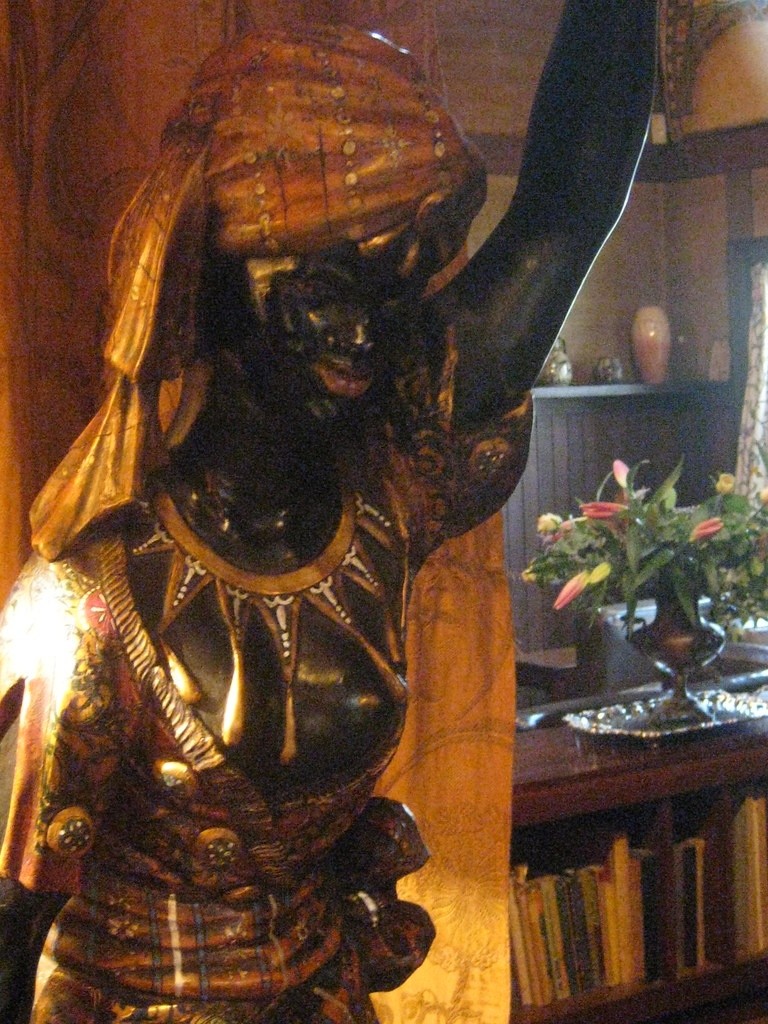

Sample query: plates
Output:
[[560, 689, 768, 738]]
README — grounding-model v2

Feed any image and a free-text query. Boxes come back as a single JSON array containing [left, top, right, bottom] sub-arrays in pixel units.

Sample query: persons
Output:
[[0, 0, 659, 1024]]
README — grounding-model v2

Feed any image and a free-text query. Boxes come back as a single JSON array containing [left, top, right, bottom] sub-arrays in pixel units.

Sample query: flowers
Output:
[[522, 441, 768, 641]]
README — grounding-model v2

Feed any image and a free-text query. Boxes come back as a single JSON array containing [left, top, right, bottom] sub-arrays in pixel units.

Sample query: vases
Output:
[[631, 307, 672, 385], [624, 585, 727, 724]]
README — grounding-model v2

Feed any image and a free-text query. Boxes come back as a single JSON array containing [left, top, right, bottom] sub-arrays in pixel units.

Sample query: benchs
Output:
[[568, 595, 713, 697]]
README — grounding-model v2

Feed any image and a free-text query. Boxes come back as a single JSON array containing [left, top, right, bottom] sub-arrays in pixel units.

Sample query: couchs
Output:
[[516, 590, 768, 729]]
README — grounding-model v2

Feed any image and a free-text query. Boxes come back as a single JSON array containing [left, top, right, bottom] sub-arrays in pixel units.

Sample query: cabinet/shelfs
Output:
[[509, 687, 768, 1024]]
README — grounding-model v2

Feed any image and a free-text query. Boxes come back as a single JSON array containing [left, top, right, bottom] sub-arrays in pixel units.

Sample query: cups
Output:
[[595, 357, 623, 384]]
